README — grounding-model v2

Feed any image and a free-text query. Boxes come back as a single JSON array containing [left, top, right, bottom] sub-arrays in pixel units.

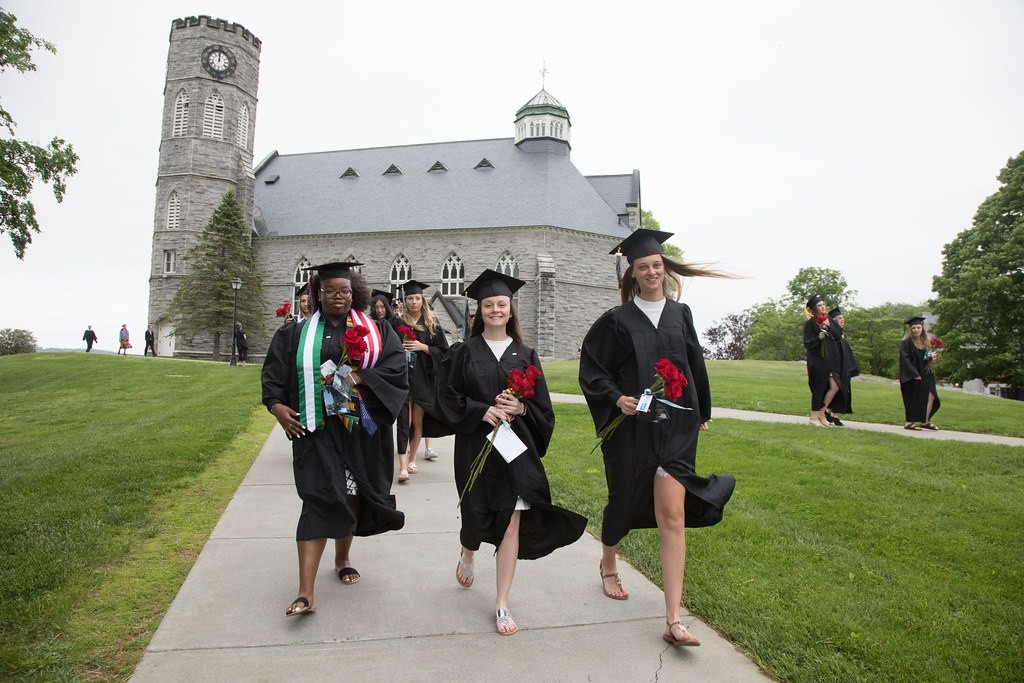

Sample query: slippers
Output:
[[921, 423, 939, 430], [455, 547, 475, 589], [905, 423, 922, 430], [496, 608, 518, 635], [339, 567, 361, 584], [286, 597, 315, 617]]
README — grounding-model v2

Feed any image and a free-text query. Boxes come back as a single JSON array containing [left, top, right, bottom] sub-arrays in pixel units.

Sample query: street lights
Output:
[[230, 273, 243, 364]]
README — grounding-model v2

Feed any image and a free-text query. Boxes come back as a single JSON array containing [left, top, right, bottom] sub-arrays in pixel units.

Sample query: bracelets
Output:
[[349, 374, 357, 385], [519, 404, 525, 415]]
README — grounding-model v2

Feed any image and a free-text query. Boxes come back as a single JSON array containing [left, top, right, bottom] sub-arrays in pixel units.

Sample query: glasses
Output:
[[320, 289, 352, 298]]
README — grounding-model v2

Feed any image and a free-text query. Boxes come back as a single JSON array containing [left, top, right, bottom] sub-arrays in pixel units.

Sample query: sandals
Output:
[[663, 621, 700, 646], [600, 560, 628, 600]]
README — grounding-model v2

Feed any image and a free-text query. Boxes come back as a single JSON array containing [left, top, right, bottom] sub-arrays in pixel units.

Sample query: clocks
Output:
[[201, 44, 237, 78]]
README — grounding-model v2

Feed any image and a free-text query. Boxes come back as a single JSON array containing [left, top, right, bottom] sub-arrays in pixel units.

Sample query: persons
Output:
[[284, 283, 310, 323], [83, 326, 97, 352], [370, 280, 475, 480], [448, 267, 590, 634], [144, 325, 157, 356], [899, 316, 940, 430], [118, 324, 129, 355], [235, 324, 248, 364], [803, 294, 859, 427], [261, 262, 409, 616], [580, 228, 736, 646]]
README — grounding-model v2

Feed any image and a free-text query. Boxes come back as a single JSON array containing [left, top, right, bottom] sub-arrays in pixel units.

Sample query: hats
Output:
[[396, 280, 430, 307], [369, 289, 393, 306], [904, 317, 926, 335], [301, 262, 364, 313], [296, 283, 309, 297], [462, 269, 526, 339], [829, 308, 841, 318], [804, 294, 825, 320], [609, 229, 674, 287]]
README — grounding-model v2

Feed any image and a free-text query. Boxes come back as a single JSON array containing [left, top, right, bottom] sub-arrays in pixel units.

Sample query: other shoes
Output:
[[830, 417, 844, 426], [810, 418, 825, 427], [824, 410, 832, 421], [819, 418, 828, 425], [398, 470, 410, 481], [408, 462, 418, 474], [153, 354, 157, 357]]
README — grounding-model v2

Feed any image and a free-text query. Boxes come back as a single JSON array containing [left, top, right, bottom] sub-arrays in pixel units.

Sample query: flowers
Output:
[[337, 325, 371, 371], [924, 337, 943, 376], [397, 325, 416, 343], [276, 303, 291, 319], [590, 359, 689, 456], [816, 315, 830, 359], [457, 363, 541, 509]]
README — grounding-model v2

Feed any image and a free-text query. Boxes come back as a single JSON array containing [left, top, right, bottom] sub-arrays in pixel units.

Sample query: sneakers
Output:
[[424, 448, 439, 459]]
